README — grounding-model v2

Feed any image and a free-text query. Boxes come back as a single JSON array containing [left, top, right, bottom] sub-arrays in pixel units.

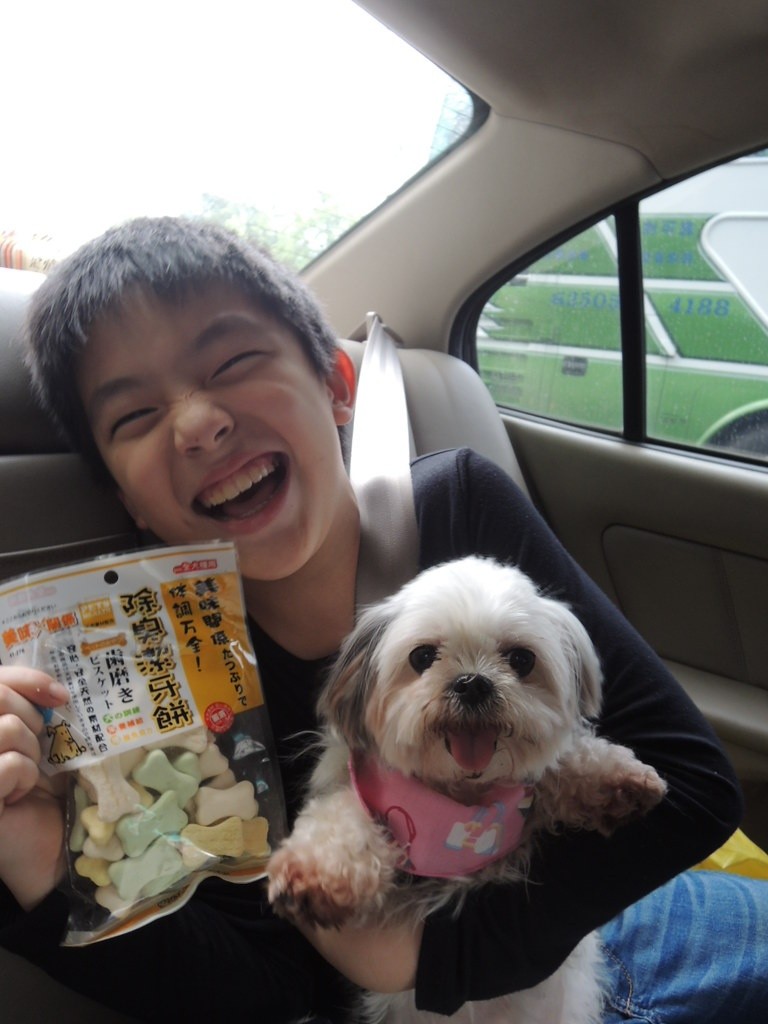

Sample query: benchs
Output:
[[0, 264, 530, 1024]]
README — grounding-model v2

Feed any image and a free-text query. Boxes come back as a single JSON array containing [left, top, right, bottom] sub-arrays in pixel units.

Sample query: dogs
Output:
[[260, 552, 673, 1024]]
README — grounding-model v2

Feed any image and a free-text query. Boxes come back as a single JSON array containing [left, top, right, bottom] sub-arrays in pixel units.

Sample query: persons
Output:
[[0, 212, 766, 1024]]
